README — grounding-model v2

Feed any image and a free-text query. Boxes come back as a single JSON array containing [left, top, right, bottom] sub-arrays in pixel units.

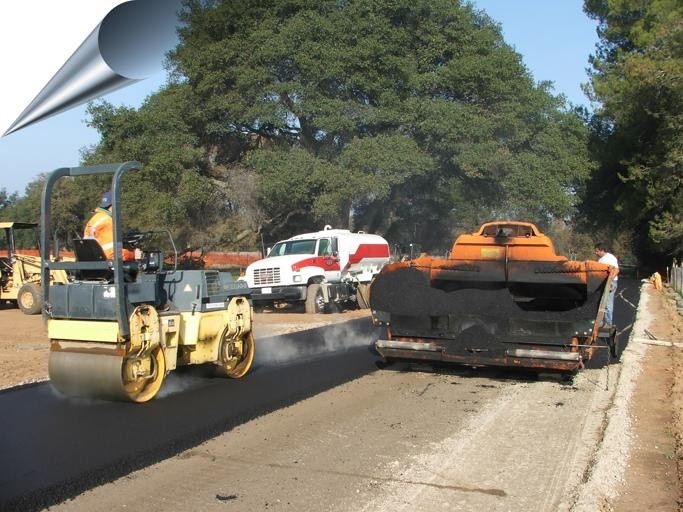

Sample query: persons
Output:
[[595, 244, 620, 329], [84, 192, 144, 261]]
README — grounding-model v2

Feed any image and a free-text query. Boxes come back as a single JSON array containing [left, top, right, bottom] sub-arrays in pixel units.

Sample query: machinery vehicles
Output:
[[369, 220, 618, 378], [235, 224, 391, 314]]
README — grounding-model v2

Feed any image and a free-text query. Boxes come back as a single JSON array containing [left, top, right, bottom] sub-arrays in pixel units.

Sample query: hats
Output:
[[100, 192, 112, 208]]
